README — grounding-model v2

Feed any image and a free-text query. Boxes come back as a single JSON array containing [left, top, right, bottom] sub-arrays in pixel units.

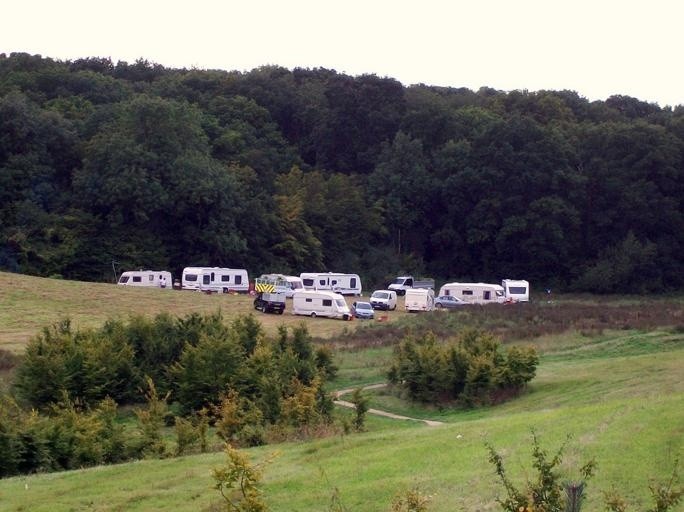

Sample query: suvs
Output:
[[253, 291, 286, 315]]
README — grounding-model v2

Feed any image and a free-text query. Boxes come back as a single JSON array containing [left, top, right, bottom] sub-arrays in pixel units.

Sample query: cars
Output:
[[350, 300, 374, 318], [435, 296, 467, 307]]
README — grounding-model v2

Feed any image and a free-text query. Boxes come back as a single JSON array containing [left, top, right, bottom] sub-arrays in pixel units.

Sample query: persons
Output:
[[544, 287, 554, 304], [159, 277, 167, 288]]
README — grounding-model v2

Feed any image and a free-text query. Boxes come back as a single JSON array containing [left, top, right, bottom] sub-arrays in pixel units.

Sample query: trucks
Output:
[[388, 276, 435, 295]]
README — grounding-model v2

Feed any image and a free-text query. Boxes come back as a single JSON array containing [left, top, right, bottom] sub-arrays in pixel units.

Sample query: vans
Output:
[[371, 290, 397, 311], [292, 290, 350, 321]]
